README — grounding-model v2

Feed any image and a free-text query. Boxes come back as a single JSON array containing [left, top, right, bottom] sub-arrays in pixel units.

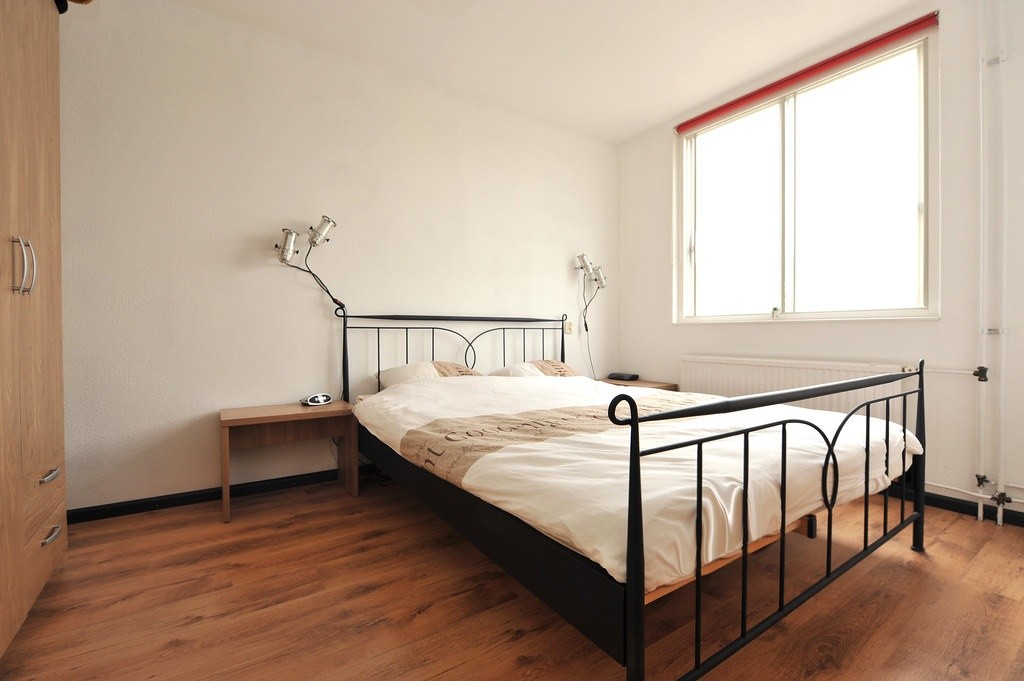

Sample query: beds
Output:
[[335, 307, 926, 681]]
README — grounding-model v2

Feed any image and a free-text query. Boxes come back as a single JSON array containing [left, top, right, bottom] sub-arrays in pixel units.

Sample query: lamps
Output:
[[576, 253, 607, 289], [274, 228, 300, 264], [308, 215, 337, 247]]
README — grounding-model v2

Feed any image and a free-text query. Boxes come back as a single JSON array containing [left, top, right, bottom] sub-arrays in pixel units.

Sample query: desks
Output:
[[596, 378, 678, 391], [220, 400, 359, 522]]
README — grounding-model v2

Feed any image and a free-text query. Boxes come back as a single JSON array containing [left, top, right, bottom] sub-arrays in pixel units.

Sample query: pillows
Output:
[[489, 359, 579, 377], [373, 361, 485, 390]]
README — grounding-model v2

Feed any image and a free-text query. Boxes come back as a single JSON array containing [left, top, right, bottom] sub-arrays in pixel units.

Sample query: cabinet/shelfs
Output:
[[0, 0, 69, 659]]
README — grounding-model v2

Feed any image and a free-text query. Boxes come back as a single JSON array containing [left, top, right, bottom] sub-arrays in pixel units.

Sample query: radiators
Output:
[[681, 356, 903, 428]]
